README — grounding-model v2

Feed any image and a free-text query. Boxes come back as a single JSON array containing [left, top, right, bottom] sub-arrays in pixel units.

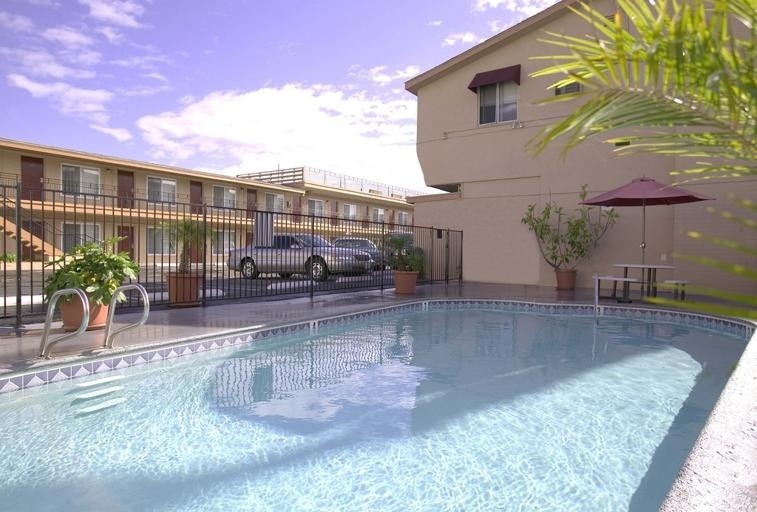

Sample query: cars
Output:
[[226, 232, 378, 284], [332, 236, 386, 272]]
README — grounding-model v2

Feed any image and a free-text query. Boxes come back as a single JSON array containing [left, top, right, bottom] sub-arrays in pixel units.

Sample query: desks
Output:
[[611, 263, 677, 304]]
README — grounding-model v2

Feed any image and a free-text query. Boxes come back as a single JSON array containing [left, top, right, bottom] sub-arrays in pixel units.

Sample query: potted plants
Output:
[[163, 215, 215, 309], [520, 184, 620, 292], [381, 230, 427, 294], [41, 235, 140, 333]]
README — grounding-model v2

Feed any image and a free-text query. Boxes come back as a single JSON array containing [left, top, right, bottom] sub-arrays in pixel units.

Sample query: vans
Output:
[[382, 230, 415, 260]]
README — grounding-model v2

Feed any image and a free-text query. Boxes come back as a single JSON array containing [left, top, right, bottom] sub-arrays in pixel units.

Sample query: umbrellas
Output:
[[576, 170, 716, 297]]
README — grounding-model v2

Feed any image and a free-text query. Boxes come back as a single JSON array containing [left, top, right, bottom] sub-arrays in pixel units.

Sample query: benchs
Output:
[[591, 273, 691, 305]]
